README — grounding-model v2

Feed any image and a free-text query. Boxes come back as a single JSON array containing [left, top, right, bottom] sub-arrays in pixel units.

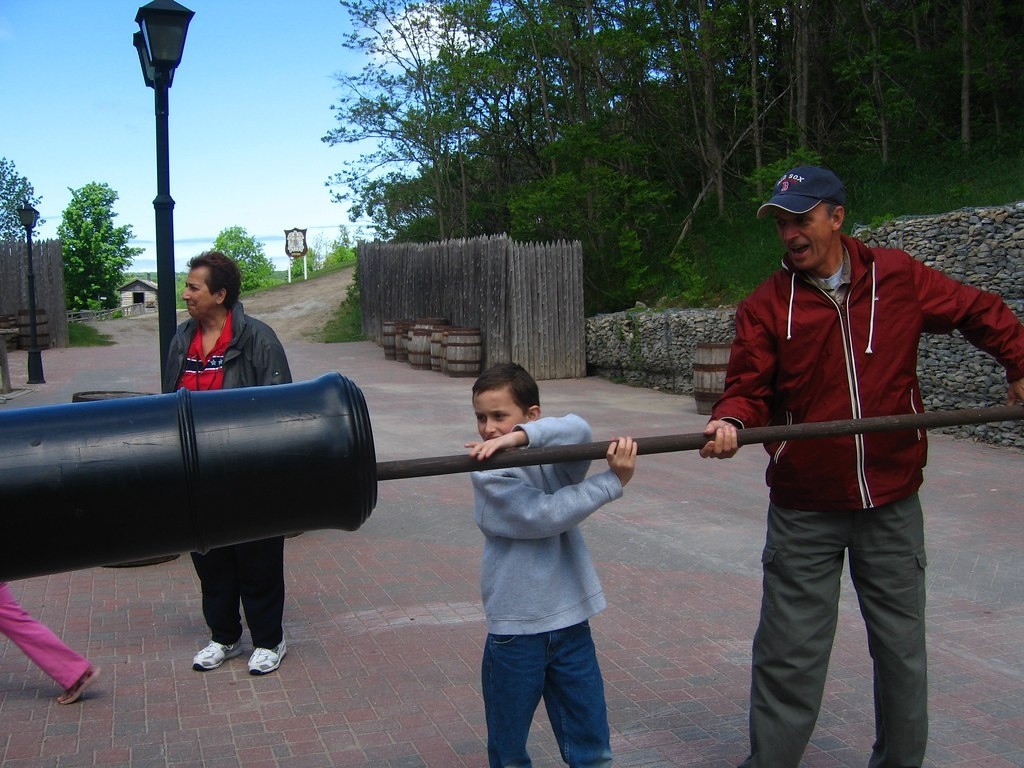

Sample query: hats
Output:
[[756, 166, 846, 218]]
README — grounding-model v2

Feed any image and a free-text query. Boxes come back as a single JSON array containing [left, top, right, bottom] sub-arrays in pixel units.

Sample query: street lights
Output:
[[131, 0, 197, 399], [16, 198, 47, 385]]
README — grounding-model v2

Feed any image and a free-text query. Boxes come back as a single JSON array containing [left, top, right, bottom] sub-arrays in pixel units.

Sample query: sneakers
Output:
[[192, 637, 242, 670], [248, 634, 286, 674]]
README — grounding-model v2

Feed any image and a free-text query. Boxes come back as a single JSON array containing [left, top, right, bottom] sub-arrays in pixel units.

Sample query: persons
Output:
[[699, 164, 1024, 768], [162, 252, 293, 676], [0, 582, 101, 705], [463, 362, 639, 768]]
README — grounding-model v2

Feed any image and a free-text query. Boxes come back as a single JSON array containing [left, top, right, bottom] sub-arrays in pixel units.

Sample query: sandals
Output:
[[59, 665, 101, 705]]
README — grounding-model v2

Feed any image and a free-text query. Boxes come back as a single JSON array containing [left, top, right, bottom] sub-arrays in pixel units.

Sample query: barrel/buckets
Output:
[[694, 342, 732, 414], [0, 309, 50, 352], [383, 318, 482, 378]]
